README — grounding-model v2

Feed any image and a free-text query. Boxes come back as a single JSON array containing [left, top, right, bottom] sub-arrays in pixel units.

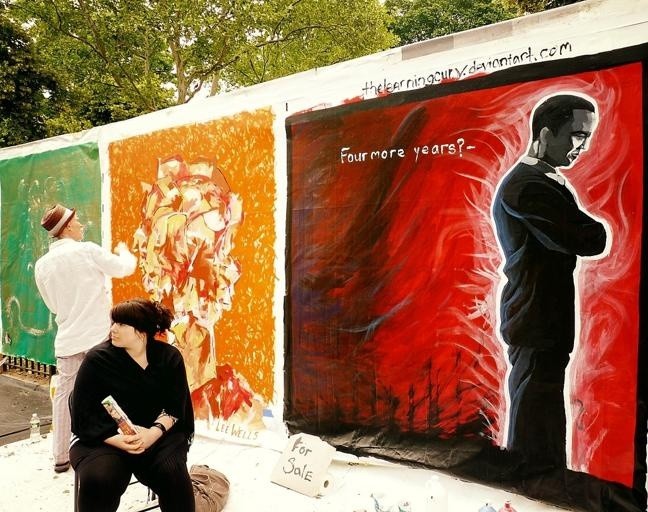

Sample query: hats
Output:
[[40, 203, 75, 239]]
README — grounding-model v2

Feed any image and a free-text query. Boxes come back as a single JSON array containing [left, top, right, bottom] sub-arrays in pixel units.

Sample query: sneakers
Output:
[[55, 461, 70, 474]]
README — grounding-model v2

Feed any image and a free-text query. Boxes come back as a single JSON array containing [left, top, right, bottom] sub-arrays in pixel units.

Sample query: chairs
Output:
[[69, 388, 157, 512]]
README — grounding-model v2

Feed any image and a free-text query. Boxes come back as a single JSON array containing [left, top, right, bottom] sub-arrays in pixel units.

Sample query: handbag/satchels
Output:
[[188, 464, 231, 512]]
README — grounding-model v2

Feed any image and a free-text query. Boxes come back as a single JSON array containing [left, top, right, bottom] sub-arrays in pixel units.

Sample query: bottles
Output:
[[29, 413, 41, 444]]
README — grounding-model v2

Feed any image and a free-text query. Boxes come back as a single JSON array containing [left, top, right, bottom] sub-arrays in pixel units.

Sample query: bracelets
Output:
[[150, 422, 167, 434]]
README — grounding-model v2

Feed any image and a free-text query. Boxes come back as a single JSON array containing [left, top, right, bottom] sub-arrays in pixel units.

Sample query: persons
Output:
[[30, 203, 137, 472], [68, 292, 198, 511], [489, 91, 606, 468]]
